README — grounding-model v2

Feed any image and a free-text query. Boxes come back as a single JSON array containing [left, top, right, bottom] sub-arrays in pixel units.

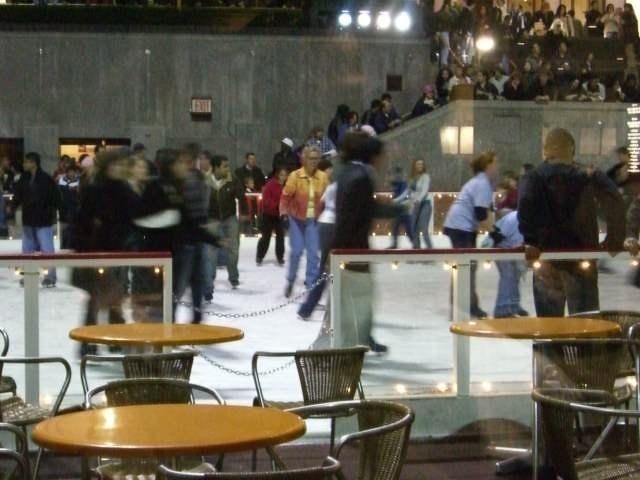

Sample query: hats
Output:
[[422, 84, 433, 93], [280, 137, 293, 147]]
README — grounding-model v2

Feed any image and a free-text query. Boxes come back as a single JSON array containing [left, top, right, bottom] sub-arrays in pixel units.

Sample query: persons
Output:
[[480, 146, 640, 319], [443, 152, 506, 320], [256, 125, 403, 357], [392, 158, 435, 266], [234, 151, 267, 236], [412, 0, 640, 118], [386, 167, 415, 249], [1, 139, 250, 364], [327, 92, 403, 144], [516, 127, 627, 373]]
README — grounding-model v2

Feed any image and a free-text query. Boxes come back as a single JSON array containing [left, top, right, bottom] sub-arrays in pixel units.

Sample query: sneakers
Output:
[[495, 308, 528, 317], [369, 338, 386, 352], [472, 306, 486, 317], [42, 278, 56, 285], [255, 256, 284, 264]]
